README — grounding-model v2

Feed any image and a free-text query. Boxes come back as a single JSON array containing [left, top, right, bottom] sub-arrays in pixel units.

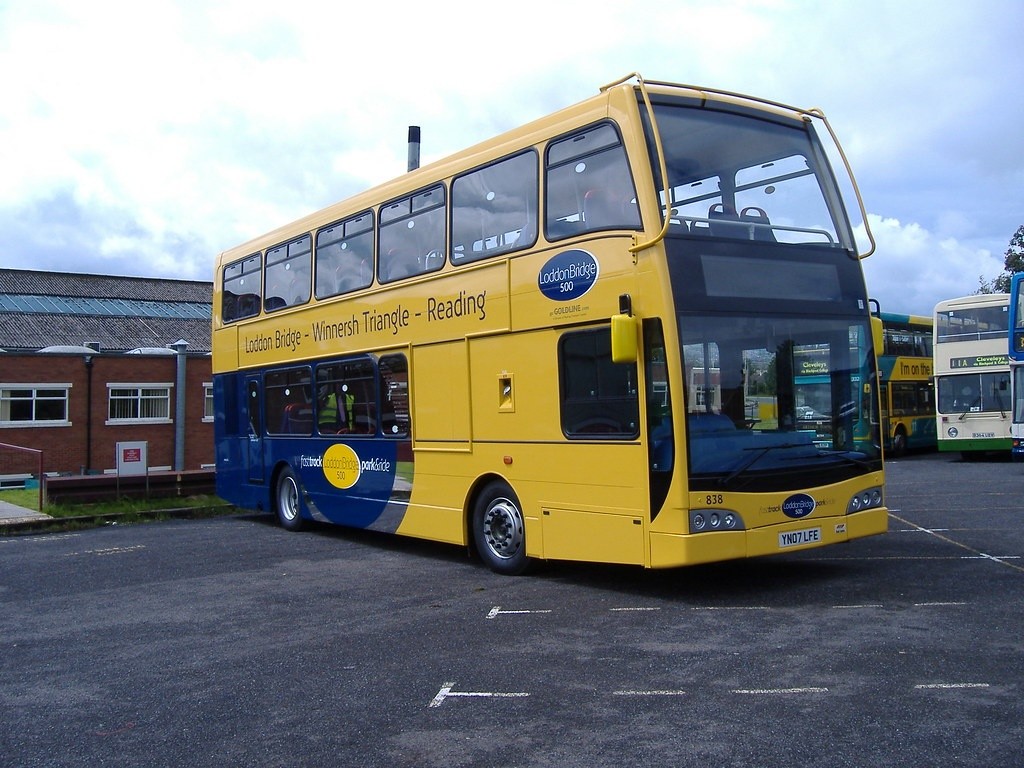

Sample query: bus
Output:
[[997, 270, 1024, 453], [209, 70, 889, 580], [925, 293, 1024, 461], [788, 310, 990, 460]]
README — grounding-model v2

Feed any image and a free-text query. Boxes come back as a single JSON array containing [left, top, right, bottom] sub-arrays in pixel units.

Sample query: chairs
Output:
[[962, 324, 978, 340], [663, 204, 776, 242], [888, 340, 921, 356], [944, 324, 961, 342], [583, 188, 643, 228], [235, 244, 485, 317], [280, 401, 312, 434]]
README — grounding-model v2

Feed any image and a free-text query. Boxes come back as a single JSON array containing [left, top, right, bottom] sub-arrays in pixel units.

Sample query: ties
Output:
[[338, 395, 346, 422]]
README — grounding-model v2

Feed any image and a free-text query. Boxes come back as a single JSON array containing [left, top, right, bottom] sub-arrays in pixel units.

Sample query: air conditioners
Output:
[[84, 342, 100, 352]]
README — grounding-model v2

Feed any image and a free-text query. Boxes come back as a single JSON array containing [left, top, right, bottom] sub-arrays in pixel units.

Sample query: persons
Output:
[[319, 381, 356, 432]]
